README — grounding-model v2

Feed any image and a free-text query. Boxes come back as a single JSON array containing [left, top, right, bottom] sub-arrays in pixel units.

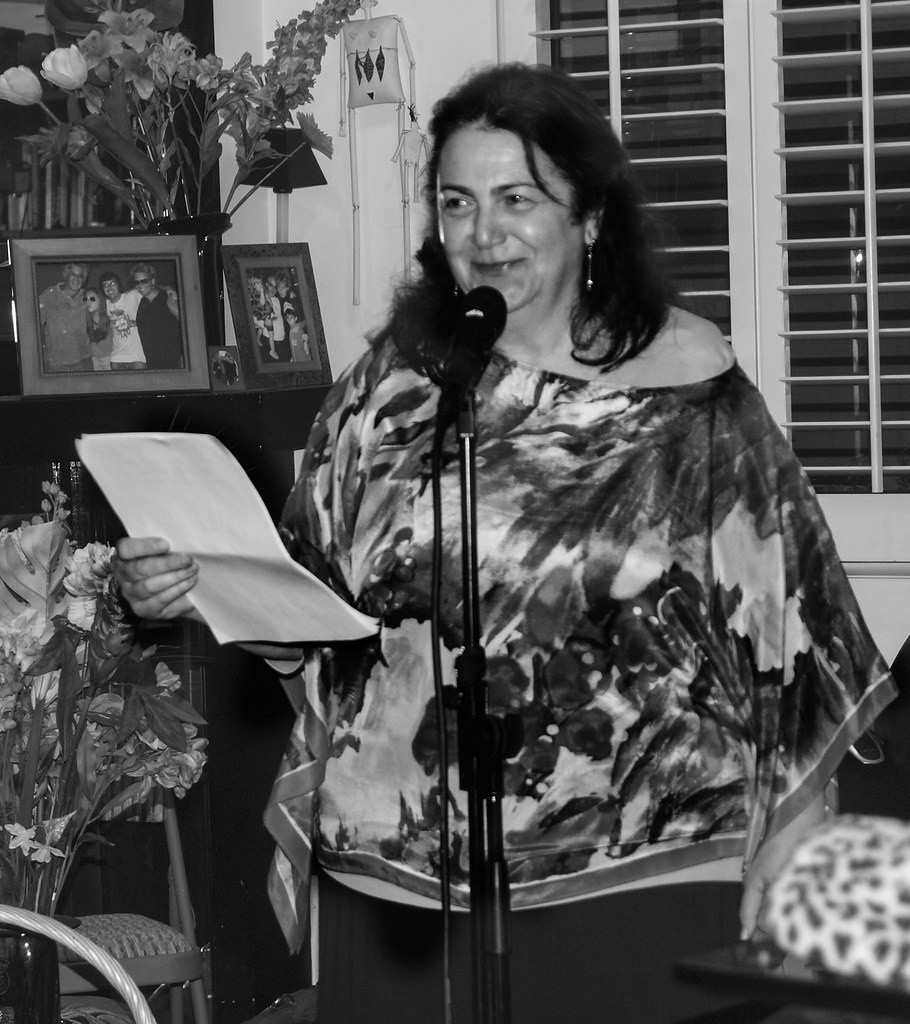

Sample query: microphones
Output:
[[436, 285, 507, 425]]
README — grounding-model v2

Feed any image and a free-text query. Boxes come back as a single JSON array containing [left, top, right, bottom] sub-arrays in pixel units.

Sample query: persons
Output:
[[246, 269, 313, 364], [108, 66, 896, 1024], [36, 260, 188, 372]]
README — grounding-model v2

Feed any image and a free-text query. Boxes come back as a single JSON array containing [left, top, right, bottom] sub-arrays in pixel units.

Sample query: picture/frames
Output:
[[220, 242, 333, 391], [11, 234, 212, 399]]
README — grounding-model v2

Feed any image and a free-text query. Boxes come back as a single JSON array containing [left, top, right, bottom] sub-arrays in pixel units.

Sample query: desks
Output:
[[673, 943, 910, 1024], [0, 385, 334, 524]]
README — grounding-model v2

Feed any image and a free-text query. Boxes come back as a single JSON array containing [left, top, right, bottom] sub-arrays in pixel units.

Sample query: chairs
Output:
[[57, 785, 209, 1024]]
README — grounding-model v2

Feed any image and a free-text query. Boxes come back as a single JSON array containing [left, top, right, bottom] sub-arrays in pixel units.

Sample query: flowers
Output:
[[0, 0, 365, 222], [0, 480, 209, 919]]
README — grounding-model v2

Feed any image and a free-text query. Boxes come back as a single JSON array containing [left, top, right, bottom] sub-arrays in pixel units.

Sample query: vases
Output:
[[9, 931, 61, 1024], [147, 213, 231, 344]]
